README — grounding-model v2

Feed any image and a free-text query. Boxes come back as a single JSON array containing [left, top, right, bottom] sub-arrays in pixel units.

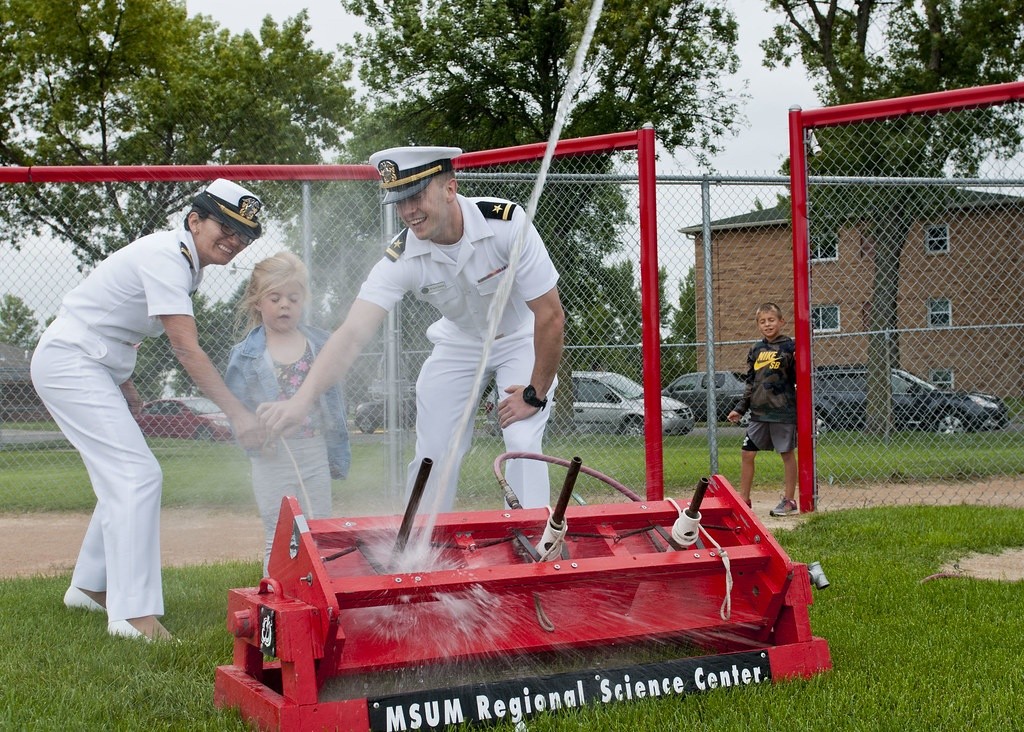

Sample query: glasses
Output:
[[207, 216, 254, 245]]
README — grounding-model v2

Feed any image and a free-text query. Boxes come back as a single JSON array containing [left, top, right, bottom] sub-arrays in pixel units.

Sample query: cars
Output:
[[482, 370, 694, 438], [133, 396, 234, 442], [660, 371, 749, 422], [355, 395, 417, 434]]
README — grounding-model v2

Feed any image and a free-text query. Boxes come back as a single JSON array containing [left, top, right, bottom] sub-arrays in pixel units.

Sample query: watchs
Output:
[[523, 385, 548, 412]]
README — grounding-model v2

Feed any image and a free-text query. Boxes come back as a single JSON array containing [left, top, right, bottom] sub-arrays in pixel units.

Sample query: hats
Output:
[[192, 178, 265, 239], [369, 146, 462, 205]]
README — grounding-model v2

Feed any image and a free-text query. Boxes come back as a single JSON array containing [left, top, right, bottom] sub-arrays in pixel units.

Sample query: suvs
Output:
[[812, 365, 1011, 435]]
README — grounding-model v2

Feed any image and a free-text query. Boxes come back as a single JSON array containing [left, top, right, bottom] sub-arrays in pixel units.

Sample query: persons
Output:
[[256, 146, 566, 515], [728, 303, 799, 517], [223, 252, 352, 591], [30, 178, 274, 645]]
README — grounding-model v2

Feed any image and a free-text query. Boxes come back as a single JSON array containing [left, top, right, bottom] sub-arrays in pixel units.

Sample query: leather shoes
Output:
[[107, 620, 196, 649], [63, 585, 106, 613]]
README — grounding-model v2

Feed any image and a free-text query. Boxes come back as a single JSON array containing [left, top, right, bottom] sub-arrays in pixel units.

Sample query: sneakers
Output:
[[770, 497, 800, 517], [744, 499, 752, 509]]
